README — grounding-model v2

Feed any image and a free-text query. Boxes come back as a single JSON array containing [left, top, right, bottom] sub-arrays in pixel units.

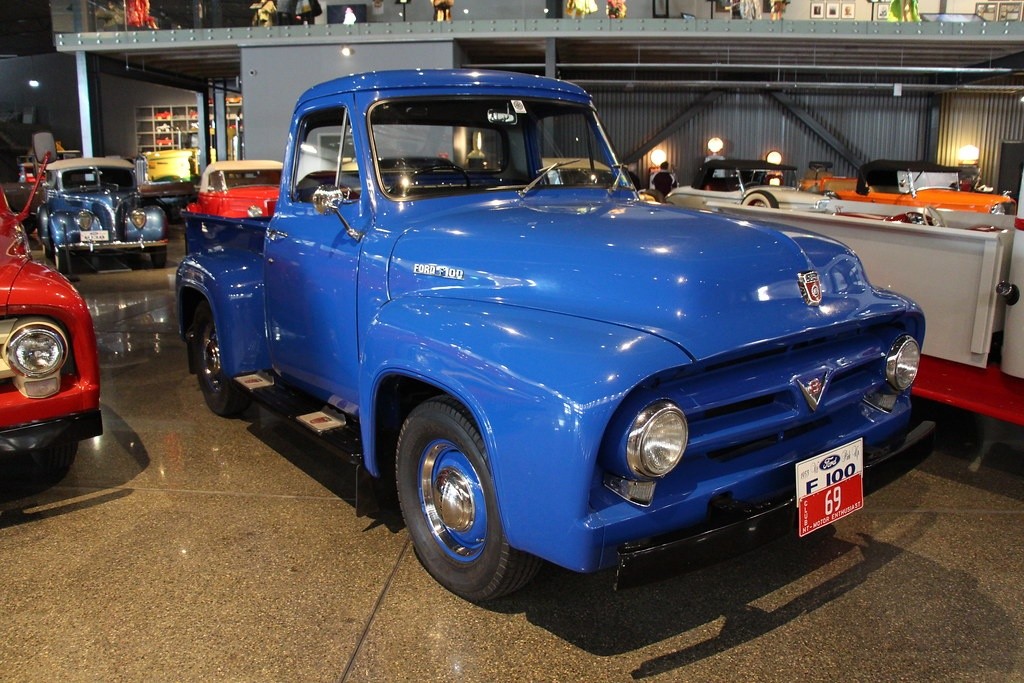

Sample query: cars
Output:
[[529, 156, 663, 206], [820, 159, 1018, 218], [665, 157, 834, 214], [1, 151, 283, 503]]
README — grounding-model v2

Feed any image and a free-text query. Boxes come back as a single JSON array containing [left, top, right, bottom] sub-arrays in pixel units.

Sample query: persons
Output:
[[651, 161, 678, 203]]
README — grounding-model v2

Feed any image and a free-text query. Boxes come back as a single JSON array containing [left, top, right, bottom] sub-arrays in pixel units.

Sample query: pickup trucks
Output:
[[174, 68, 926, 606]]
